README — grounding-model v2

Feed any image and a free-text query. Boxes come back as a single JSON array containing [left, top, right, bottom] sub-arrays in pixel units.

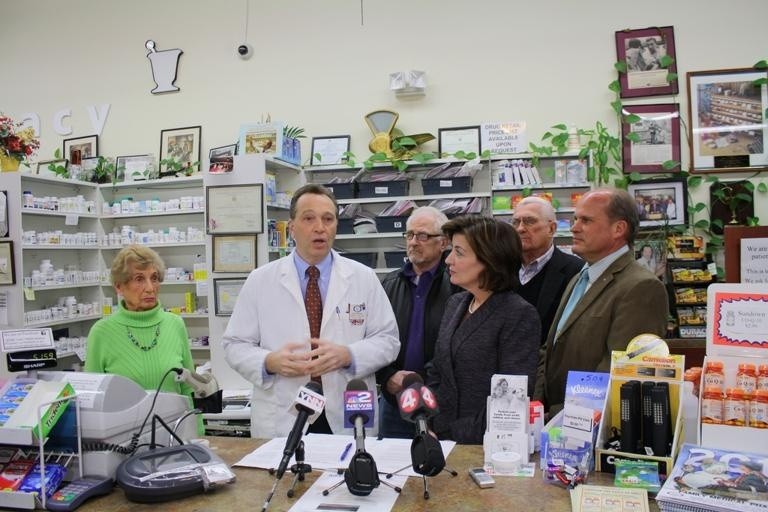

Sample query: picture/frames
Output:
[[158, 125, 202, 179], [613, 24, 768, 241], [61, 135, 100, 183], [310, 134, 352, 166], [438, 125, 483, 161], [238, 121, 283, 159]]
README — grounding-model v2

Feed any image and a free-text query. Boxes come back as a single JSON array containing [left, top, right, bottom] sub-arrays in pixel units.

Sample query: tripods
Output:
[[323, 467, 402, 495], [385, 464, 459, 500], [267, 465, 343, 499]]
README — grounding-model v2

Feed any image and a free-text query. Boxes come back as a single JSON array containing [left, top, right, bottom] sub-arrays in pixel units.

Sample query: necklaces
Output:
[[468, 296, 475, 314], [126, 324, 161, 351]]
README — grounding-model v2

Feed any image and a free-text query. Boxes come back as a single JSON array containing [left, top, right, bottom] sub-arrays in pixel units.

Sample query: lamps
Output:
[[388, 70, 427, 99]]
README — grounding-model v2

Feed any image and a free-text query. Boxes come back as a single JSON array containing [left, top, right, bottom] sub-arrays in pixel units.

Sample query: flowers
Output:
[[0, 112, 41, 169]]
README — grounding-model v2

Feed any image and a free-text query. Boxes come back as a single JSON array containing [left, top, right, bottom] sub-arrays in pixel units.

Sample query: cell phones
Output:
[[469, 466, 495, 490]]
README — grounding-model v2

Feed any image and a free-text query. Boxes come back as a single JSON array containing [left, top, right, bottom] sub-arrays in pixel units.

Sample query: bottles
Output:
[[21, 190, 95, 216], [749, 391, 768, 428], [735, 363, 756, 392], [104, 224, 207, 246], [55, 336, 86, 356], [757, 363, 768, 390], [704, 361, 726, 389], [725, 389, 748, 426], [23, 229, 99, 247], [701, 389, 724, 424], [57, 295, 100, 320], [23, 258, 81, 289], [102, 195, 207, 215]]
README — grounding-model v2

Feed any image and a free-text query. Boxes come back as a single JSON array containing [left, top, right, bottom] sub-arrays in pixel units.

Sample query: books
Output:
[[654, 442, 767, 512]]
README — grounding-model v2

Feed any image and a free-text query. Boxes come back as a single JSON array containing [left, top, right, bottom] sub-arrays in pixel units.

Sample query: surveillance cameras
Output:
[[236, 41, 254, 62]]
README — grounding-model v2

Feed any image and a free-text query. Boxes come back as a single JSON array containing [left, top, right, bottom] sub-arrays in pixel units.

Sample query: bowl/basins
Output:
[[490, 451, 522, 473]]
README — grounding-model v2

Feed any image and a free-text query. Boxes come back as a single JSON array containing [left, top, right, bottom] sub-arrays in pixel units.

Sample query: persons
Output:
[[83, 244, 205, 435], [533, 186, 670, 424], [657, 127, 665, 144], [635, 195, 676, 220], [512, 195, 586, 347], [649, 123, 657, 144], [498, 378, 512, 402], [625, 39, 654, 71], [636, 245, 656, 275], [222, 184, 402, 438], [390, 212, 542, 445], [375, 205, 467, 441], [491, 384, 509, 409], [646, 37, 668, 71], [168, 140, 193, 163]]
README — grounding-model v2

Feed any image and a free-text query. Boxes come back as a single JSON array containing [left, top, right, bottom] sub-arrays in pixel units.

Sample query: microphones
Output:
[[343, 380, 377, 451], [395, 373, 446, 435], [274, 379, 328, 477]]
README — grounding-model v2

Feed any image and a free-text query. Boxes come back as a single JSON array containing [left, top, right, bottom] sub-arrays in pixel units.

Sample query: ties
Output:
[[553, 269, 589, 347], [305, 266, 322, 385]]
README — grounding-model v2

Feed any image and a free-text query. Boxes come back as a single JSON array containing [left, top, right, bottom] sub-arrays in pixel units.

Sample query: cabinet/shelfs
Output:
[[0, 395, 86, 512], [664, 251, 720, 342]]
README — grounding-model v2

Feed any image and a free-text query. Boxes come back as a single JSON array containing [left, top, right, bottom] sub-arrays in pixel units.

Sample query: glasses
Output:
[[403, 233, 447, 241]]
